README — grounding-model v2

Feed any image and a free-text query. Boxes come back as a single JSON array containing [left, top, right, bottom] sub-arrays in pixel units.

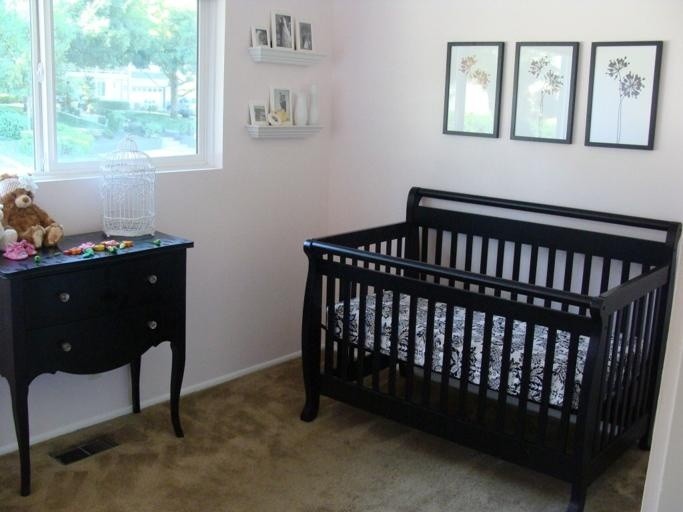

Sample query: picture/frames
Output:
[[583, 39, 664, 151], [269, 10, 296, 52], [248, 99, 269, 127], [268, 85, 294, 128], [294, 15, 316, 54], [509, 39, 581, 145], [441, 38, 507, 140], [248, 24, 273, 47]]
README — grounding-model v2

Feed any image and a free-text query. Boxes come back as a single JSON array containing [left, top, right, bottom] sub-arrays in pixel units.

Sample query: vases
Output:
[[294, 90, 310, 127], [308, 80, 322, 128]]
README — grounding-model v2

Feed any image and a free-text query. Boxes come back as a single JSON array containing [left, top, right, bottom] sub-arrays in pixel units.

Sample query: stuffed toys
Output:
[[0, 205, 18, 252], [0, 171, 64, 249]]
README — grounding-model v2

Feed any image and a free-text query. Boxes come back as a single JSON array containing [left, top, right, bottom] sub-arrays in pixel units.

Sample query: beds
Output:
[[299, 185, 683, 512]]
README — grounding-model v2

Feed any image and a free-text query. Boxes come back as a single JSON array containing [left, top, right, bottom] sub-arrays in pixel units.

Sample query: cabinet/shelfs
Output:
[[246, 47, 324, 137], [0, 229, 196, 497]]
